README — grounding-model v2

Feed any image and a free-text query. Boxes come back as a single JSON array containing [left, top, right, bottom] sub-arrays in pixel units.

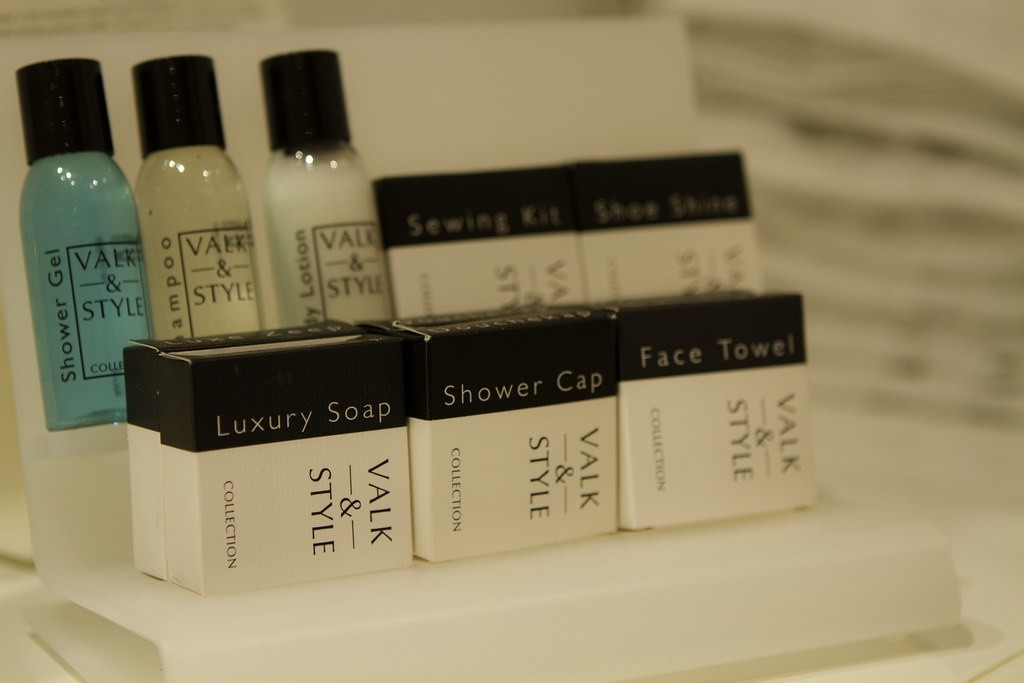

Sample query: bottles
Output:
[[13, 53, 151, 432], [131, 55, 264, 339], [262, 47, 392, 324]]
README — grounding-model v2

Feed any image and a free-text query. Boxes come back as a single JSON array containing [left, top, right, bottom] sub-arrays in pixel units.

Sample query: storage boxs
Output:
[[123, 150, 816, 594]]
